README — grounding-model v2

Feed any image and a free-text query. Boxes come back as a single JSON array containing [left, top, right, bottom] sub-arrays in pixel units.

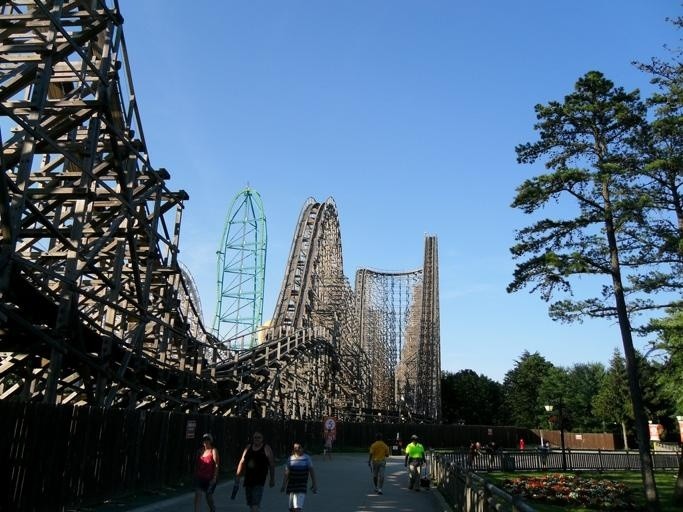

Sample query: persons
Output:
[[541, 440, 551, 466], [192, 432, 219, 512], [232, 428, 275, 512], [366, 432, 390, 494], [403, 434, 426, 492], [321, 428, 332, 461], [279, 439, 319, 512]]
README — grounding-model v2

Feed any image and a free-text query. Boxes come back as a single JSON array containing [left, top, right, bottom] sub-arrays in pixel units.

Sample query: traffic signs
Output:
[[323, 417, 336, 433]]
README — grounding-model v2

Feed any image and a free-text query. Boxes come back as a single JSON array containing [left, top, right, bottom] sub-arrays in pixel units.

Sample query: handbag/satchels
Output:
[[231, 481, 240, 500], [420, 461, 431, 490]]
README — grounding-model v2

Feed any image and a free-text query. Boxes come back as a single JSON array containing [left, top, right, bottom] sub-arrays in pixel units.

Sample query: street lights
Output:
[[543, 394, 568, 471]]
